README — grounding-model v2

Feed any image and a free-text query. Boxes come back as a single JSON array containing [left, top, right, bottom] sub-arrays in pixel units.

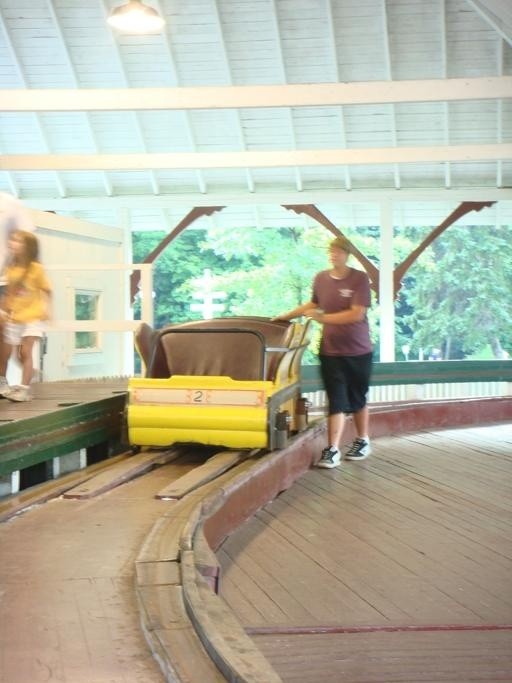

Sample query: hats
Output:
[[330, 234, 350, 251]]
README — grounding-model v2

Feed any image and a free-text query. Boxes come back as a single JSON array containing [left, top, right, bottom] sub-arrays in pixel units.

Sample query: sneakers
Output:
[[317, 443, 342, 469], [5, 385, 32, 402], [0, 376, 11, 398], [344, 435, 371, 461]]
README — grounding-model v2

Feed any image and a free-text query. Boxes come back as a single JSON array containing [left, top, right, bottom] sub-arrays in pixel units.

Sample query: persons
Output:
[[0, 229, 58, 401], [268, 234, 374, 469], [1, 213, 19, 397]]
